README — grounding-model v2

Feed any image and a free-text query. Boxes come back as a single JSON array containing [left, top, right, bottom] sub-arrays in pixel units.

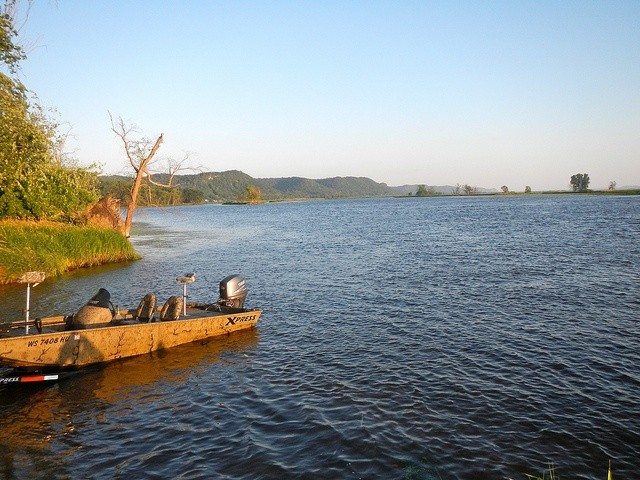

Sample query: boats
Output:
[[2, 273, 264, 379]]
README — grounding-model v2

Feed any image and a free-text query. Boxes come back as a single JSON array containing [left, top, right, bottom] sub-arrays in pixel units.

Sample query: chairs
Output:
[[120, 292, 156, 324], [160, 296, 182, 321]]
